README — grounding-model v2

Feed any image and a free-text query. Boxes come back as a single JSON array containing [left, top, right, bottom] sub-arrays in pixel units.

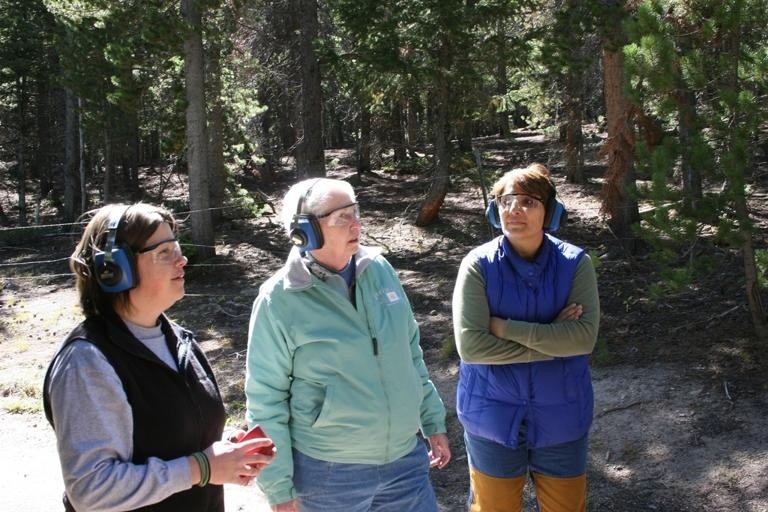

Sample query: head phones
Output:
[[484, 194, 569, 234], [289, 175, 325, 251], [91, 205, 140, 294]]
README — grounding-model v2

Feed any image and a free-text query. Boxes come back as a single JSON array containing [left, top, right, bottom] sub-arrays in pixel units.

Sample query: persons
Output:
[[451, 163, 601, 512], [43, 202, 277, 512], [245, 177, 451, 512]]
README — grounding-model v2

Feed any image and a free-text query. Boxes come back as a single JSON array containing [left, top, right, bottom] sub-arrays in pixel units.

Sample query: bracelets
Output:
[[191, 451, 210, 488]]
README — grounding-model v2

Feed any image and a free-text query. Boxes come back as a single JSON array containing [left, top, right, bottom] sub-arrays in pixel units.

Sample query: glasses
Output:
[[494, 192, 545, 211], [134, 237, 183, 264], [318, 201, 361, 227]]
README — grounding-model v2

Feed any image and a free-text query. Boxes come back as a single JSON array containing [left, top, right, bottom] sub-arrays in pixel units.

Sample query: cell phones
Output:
[[236, 424, 275, 467]]
